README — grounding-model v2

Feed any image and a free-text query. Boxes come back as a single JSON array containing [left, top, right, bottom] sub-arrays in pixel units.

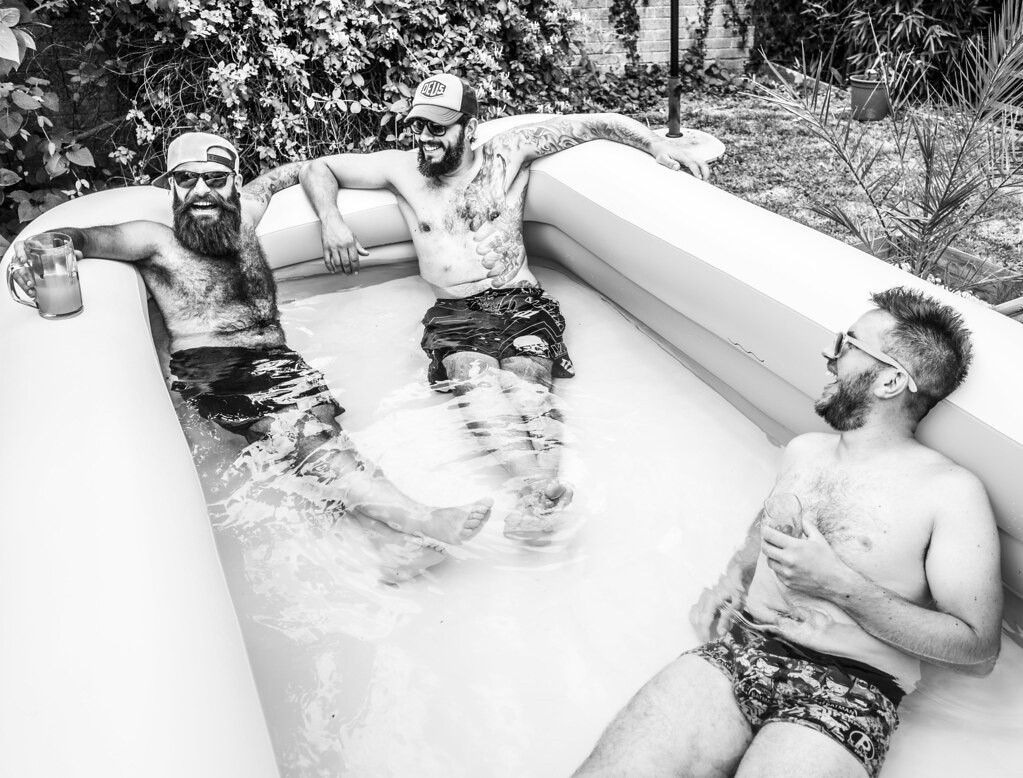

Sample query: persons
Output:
[[6, 131, 315, 364], [760, 282, 1005, 664], [295, 73, 712, 292]]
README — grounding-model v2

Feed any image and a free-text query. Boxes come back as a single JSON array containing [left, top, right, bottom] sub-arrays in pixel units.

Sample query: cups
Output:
[[5, 230, 86, 319]]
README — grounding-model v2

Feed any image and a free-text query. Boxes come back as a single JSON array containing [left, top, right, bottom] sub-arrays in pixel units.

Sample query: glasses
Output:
[[829, 330, 919, 396], [166, 170, 236, 190], [406, 120, 465, 137]]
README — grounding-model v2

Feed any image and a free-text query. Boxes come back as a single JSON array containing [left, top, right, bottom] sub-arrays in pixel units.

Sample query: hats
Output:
[[403, 73, 478, 126], [150, 131, 240, 191]]
